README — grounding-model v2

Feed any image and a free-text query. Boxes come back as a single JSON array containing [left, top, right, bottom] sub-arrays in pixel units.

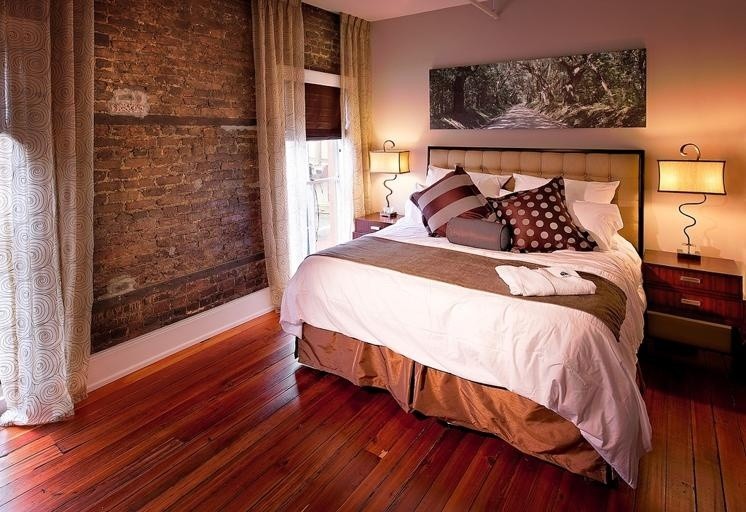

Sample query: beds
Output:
[[277, 147, 653, 458]]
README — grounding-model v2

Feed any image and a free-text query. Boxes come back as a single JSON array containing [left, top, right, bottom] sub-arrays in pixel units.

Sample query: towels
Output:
[[492, 262, 598, 299]]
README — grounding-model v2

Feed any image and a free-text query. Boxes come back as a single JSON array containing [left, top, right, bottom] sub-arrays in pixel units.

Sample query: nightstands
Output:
[[351, 211, 404, 241], [641, 247, 746, 390]]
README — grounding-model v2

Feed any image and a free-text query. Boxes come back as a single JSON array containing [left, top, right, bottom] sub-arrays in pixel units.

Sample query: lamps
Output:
[[656, 142, 727, 262], [368, 139, 409, 221]]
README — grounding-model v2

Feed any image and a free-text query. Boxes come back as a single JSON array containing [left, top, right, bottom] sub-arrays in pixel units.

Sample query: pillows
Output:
[[409, 165, 500, 245], [568, 199, 624, 252], [445, 217, 513, 252], [407, 180, 500, 233], [486, 174, 596, 256], [513, 170, 620, 204], [422, 161, 511, 190]]
